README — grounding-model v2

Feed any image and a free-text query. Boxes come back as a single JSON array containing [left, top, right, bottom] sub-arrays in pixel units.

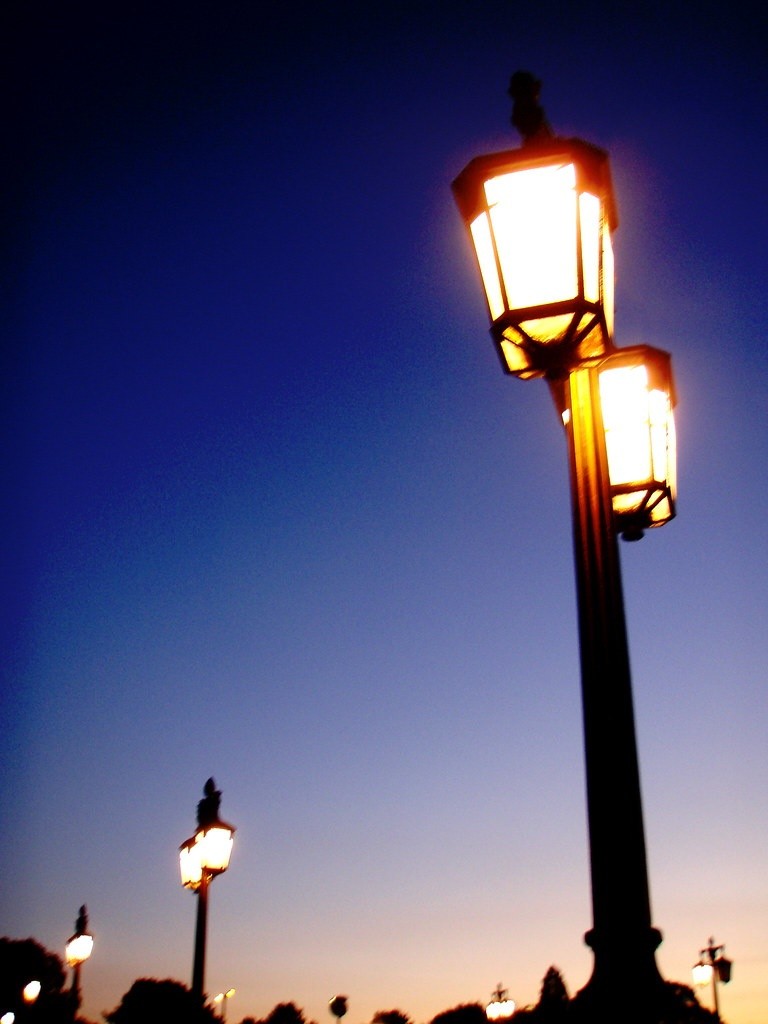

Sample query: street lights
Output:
[[177, 777, 236, 1024], [692, 937, 732, 1016], [65, 904, 94, 1024], [214, 988, 235, 1022], [485, 982, 515, 1024], [447, 74, 678, 1024]]
